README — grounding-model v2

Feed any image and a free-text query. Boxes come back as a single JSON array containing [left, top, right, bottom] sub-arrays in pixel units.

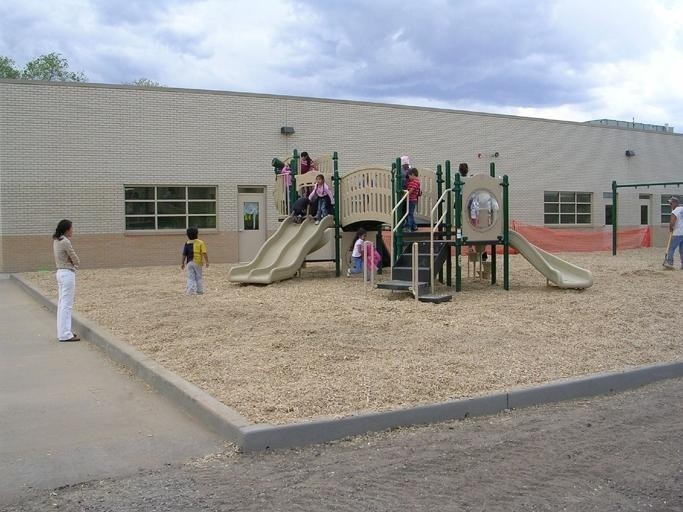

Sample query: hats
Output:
[[400, 155, 410, 165]]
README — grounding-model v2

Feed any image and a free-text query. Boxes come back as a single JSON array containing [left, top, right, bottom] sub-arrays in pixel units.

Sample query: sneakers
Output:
[[186, 291, 205, 296], [294, 216, 320, 225], [346, 268, 352, 279], [402, 222, 418, 230]]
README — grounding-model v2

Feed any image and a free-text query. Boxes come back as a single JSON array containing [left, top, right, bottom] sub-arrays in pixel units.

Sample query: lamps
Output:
[[625, 149, 635, 157], [280, 126, 295, 134]]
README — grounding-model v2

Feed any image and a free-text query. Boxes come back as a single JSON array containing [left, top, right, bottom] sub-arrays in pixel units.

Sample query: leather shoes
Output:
[[59, 333, 81, 341]]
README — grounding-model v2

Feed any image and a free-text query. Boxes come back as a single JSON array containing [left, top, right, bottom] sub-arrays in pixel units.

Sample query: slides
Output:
[[225, 214, 334, 284], [508, 229, 594, 288]]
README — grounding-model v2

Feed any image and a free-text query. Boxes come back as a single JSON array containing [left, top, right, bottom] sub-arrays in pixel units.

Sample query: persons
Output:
[[663, 197, 683, 270], [292, 197, 309, 224], [53, 219, 81, 342], [308, 175, 336, 225], [282, 164, 292, 215], [347, 228, 370, 281], [401, 168, 420, 231], [181, 227, 209, 295], [400, 156, 414, 194], [300, 152, 313, 193]]
